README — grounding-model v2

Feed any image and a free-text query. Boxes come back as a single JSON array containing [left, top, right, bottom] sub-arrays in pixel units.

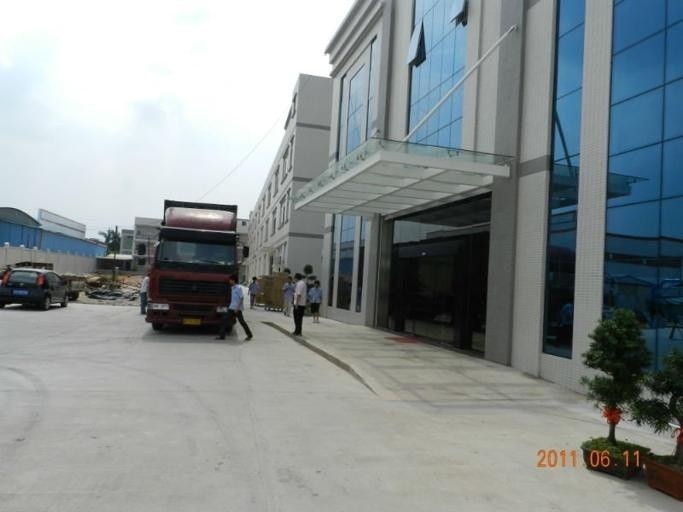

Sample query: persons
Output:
[[215, 275, 254, 340], [138, 272, 150, 313], [307, 280, 324, 324], [247, 275, 259, 309], [282, 275, 296, 316], [292, 273, 308, 335]]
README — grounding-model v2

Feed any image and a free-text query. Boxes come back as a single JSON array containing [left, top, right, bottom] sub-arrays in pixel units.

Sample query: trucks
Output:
[[1, 262, 86, 302], [138, 200, 250, 331]]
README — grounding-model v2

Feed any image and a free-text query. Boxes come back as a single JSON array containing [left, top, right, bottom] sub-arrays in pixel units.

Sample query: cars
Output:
[[0, 267, 72, 310]]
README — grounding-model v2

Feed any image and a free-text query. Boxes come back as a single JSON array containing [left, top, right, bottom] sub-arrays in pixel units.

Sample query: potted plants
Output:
[[577, 308, 682, 501]]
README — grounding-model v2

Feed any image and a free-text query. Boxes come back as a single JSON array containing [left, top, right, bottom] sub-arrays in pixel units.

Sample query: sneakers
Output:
[[217, 336, 225, 339], [293, 332, 303, 336], [245, 336, 253, 340]]
